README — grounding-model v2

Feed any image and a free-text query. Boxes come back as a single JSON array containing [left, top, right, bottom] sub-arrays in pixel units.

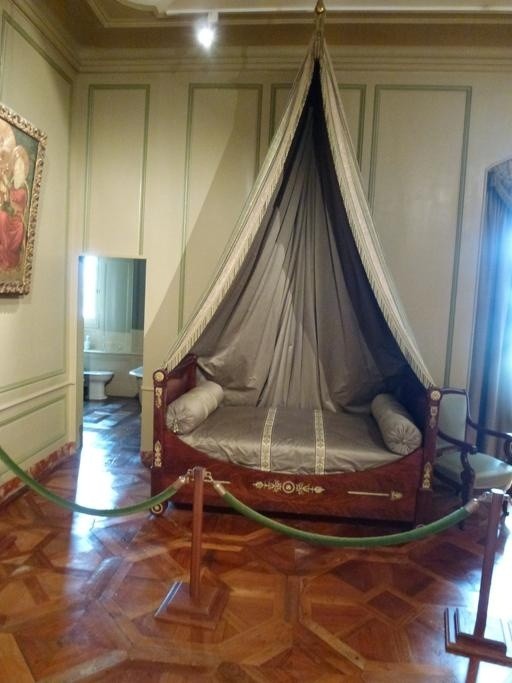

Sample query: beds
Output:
[[151, 354, 442, 531]]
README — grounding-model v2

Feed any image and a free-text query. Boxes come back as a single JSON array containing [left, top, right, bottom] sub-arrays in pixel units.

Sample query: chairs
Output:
[[434, 388, 512, 531]]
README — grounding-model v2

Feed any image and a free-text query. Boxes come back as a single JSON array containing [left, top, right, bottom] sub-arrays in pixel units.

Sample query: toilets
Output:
[[84, 370, 114, 400]]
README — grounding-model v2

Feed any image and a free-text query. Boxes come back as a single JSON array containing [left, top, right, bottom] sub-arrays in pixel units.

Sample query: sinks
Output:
[[129, 367, 143, 389]]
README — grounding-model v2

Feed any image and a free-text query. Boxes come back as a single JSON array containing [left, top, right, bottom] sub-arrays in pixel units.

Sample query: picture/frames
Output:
[[0, 103, 48, 296]]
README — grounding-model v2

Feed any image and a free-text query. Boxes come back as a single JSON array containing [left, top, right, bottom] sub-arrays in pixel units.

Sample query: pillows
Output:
[[165, 381, 225, 434], [370, 392, 422, 455]]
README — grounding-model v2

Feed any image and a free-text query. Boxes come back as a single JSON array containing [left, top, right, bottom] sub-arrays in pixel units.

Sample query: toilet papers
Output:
[[85, 335, 91, 350]]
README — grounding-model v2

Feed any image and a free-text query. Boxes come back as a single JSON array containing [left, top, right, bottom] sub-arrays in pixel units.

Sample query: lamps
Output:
[[195, 12, 219, 49]]
